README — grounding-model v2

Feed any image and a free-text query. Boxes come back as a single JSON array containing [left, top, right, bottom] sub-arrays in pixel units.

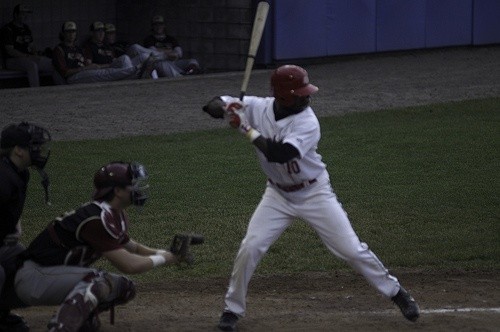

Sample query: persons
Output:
[[0, 120, 52, 332], [0, 3, 60, 87], [53, 20, 114, 84], [14, 160, 175, 332], [83, 21, 166, 80], [144, 15, 200, 76], [202, 64, 420, 332]]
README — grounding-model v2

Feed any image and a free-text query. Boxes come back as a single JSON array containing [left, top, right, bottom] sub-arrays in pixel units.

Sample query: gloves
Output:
[[223, 97, 261, 142]]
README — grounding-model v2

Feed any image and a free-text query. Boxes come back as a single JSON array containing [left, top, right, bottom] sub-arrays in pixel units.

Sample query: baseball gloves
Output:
[[169, 231, 205, 265]]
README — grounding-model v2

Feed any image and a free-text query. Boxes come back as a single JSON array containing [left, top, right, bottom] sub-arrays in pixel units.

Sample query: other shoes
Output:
[[0, 318, 30, 332], [138, 52, 154, 80]]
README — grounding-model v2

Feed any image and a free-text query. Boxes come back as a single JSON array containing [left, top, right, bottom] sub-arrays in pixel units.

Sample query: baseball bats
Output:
[[238, 2, 269, 104]]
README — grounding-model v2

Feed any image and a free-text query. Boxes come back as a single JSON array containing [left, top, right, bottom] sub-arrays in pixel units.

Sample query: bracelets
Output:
[[149, 255, 166, 268]]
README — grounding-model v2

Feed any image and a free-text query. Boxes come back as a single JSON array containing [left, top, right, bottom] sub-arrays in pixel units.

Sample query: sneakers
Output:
[[392, 285, 420, 321], [216, 311, 240, 332]]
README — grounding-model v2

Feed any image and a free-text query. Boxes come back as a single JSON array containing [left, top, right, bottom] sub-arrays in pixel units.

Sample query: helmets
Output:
[[271, 64, 319, 98], [91, 164, 128, 200]]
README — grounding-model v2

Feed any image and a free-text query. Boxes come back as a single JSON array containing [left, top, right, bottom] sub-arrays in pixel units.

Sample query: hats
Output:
[[0, 124, 27, 151], [105, 24, 116, 34], [91, 21, 107, 30], [14, 3, 32, 13], [60, 21, 77, 31], [151, 15, 165, 24]]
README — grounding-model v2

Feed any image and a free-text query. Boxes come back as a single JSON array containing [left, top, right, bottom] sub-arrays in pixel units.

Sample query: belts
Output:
[[268, 178, 317, 192]]
